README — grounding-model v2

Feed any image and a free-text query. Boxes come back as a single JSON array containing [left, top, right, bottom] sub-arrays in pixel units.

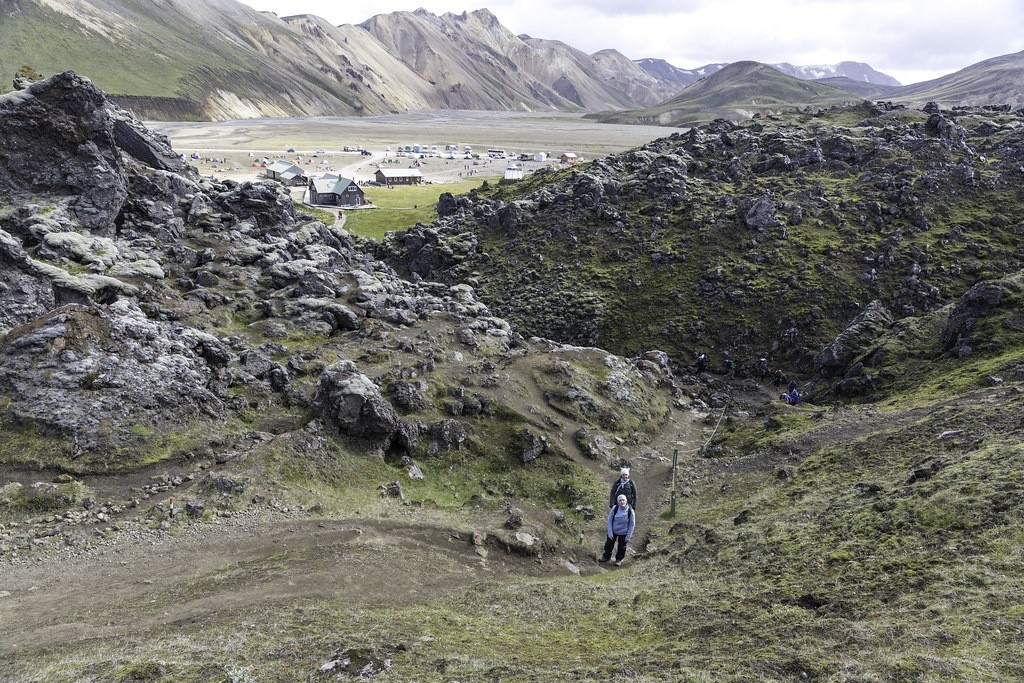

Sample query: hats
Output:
[[620, 467, 630, 476]]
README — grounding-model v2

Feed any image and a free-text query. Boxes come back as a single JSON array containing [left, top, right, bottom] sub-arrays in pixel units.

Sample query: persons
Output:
[[598, 494, 635, 566], [610, 467, 639, 511], [692, 349, 802, 409], [182, 141, 584, 220]]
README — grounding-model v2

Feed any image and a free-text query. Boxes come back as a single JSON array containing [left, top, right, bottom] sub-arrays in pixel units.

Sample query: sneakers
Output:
[[599, 556, 611, 562], [614, 559, 622, 567]]
